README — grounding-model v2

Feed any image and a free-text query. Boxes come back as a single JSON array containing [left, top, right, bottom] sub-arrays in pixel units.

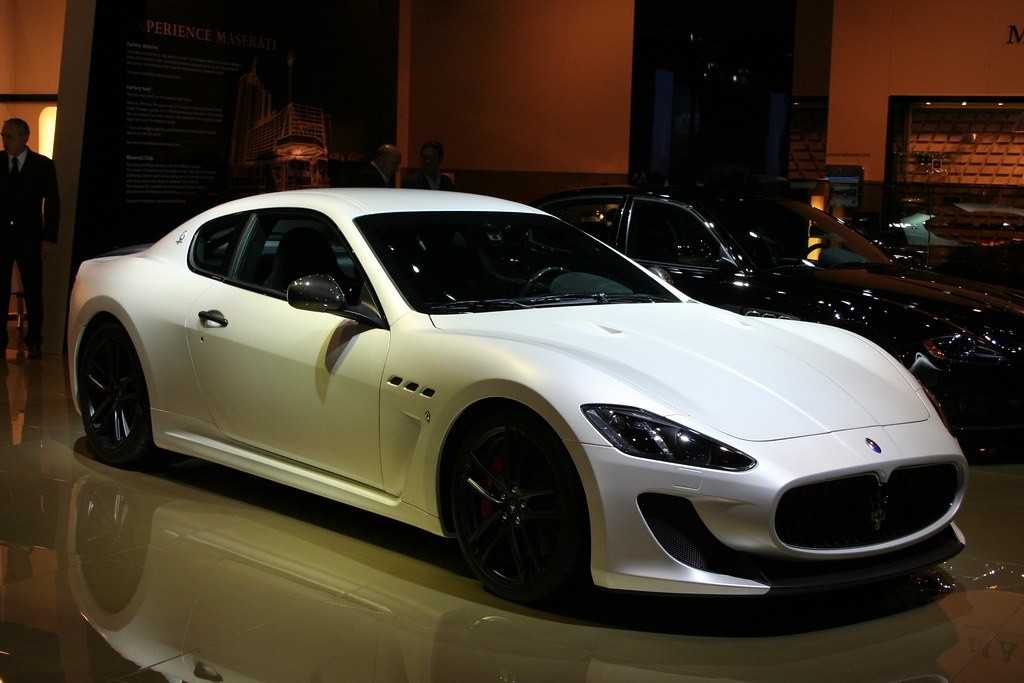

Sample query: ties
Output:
[[9, 157, 19, 190]]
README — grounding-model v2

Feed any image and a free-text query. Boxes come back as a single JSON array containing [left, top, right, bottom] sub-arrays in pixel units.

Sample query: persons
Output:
[[0, 118, 61, 357], [400, 141, 456, 191], [355, 145, 402, 187]]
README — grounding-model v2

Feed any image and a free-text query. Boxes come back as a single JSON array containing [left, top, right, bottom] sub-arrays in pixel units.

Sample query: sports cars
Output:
[[64, 182, 970, 620]]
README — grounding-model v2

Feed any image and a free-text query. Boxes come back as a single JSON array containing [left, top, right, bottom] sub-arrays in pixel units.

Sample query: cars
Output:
[[477, 183, 1024, 460]]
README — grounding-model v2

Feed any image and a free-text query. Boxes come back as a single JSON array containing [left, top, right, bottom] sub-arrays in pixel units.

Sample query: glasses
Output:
[[1, 133, 13, 140]]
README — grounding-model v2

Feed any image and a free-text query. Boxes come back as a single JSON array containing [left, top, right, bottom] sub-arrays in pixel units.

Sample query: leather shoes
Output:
[[27, 346, 42, 359], [0, 347, 6, 358]]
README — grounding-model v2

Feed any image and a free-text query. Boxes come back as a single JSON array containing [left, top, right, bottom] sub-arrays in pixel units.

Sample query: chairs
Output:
[[266, 228, 350, 297]]
